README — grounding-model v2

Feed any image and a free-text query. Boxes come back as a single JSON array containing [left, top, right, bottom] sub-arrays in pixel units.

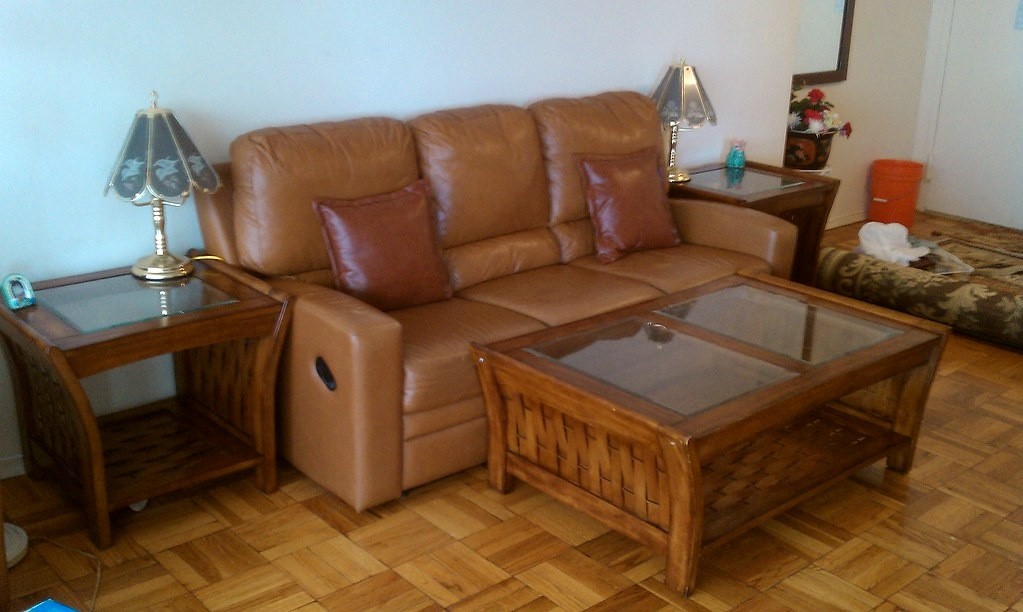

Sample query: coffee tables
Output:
[[469, 269, 952, 595]]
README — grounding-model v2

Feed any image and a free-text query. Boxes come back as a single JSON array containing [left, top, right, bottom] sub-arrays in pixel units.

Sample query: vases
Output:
[[784, 127, 836, 171]]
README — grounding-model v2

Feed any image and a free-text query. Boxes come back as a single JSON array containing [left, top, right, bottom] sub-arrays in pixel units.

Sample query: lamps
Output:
[[652, 59, 718, 184], [102, 91, 223, 281]]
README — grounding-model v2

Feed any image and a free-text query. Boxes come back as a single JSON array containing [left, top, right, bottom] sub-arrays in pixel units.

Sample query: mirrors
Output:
[[791, 0, 857, 87]]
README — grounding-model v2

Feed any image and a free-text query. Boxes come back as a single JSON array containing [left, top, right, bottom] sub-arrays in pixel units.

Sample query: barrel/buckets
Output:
[[868, 158, 924, 229]]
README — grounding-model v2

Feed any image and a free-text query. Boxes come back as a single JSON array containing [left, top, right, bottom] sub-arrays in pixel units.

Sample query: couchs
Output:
[[180, 89, 798, 514]]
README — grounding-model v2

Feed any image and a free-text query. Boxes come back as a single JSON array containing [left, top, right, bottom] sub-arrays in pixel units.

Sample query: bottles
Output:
[[725, 140, 747, 169]]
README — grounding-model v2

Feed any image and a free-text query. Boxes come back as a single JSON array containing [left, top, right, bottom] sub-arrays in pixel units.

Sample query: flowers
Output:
[[787, 78, 853, 140]]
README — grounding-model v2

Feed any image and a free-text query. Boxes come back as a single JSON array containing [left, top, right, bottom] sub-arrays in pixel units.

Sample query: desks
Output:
[[669, 159, 840, 284], [1, 248, 295, 547]]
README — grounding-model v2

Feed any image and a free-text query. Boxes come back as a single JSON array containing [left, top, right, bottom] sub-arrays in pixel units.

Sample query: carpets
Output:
[[817, 213, 1023, 354]]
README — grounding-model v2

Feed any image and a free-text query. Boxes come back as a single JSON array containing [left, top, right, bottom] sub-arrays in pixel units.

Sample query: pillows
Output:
[[570, 145, 683, 263], [313, 179, 455, 308]]
